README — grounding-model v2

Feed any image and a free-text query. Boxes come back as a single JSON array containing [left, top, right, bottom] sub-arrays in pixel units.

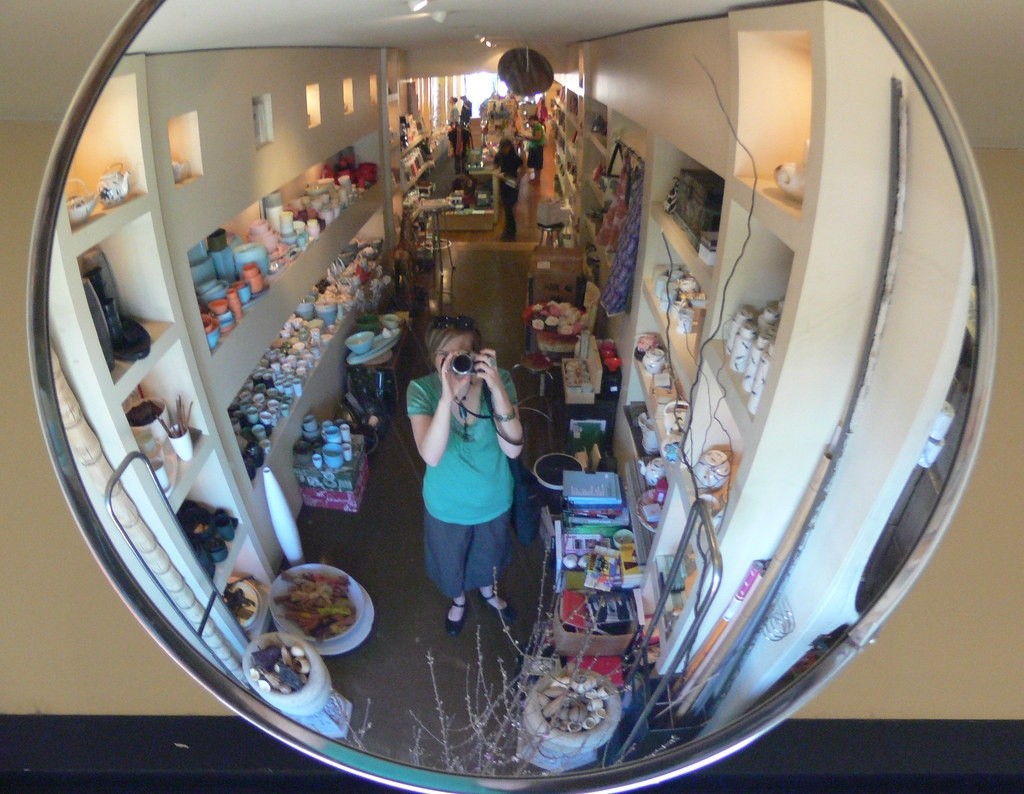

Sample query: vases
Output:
[[242, 629, 332, 716], [524, 666, 622, 755]]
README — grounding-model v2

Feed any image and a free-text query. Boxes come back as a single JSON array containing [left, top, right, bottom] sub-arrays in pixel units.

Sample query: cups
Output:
[[227, 312, 324, 480], [312, 454, 322, 469], [167, 424, 194, 461], [337, 175, 352, 191], [149, 457, 171, 493], [725, 301, 784, 415], [340, 424, 352, 442], [342, 443, 352, 462], [303, 415, 317, 431]]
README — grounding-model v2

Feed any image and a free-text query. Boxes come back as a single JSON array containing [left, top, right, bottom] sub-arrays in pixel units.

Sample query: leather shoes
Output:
[[478, 586, 512, 620], [447, 601, 466, 636]]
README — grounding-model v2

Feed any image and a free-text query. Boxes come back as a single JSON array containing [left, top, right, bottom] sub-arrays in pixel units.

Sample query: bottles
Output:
[[642, 348, 665, 374]]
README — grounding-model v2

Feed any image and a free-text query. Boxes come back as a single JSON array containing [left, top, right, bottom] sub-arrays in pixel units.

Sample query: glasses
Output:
[[433, 314, 478, 331]]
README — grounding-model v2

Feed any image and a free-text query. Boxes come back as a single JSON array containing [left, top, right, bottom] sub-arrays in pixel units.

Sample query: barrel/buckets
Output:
[[533, 452, 583, 515]]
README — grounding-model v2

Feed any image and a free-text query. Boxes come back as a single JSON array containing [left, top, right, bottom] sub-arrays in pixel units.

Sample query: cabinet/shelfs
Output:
[[556, 6, 999, 738], [53, 42, 430, 691]]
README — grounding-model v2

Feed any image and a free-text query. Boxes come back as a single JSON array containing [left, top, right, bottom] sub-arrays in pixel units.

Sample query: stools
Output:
[[511, 352, 558, 401], [529, 452, 582, 543], [422, 198, 455, 276], [537, 222, 566, 249]]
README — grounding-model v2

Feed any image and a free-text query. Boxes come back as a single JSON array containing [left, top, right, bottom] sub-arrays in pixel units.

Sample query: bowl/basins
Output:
[[344, 331, 374, 355], [322, 443, 344, 468], [613, 528, 634, 549], [294, 294, 338, 326], [381, 314, 399, 329], [321, 421, 342, 444], [301, 424, 321, 440], [187, 179, 348, 350]]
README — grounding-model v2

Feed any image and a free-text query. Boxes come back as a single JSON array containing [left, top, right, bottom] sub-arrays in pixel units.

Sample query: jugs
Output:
[[96, 161, 132, 208], [638, 457, 666, 487], [65, 178, 98, 227]]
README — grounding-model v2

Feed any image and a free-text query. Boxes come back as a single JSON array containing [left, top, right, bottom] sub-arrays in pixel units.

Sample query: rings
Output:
[[488, 358, 496, 367]]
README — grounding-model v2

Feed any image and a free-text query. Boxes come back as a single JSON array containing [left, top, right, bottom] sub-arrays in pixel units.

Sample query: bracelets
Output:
[[493, 407, 516, 422]]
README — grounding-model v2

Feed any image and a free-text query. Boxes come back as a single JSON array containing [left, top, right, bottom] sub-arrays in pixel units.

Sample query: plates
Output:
[[635, 489, 667, 533], [269, 582, 375, 656], [267, 563, 364, 642], [346, 328, 403, 365], [223, 576, 262, 632]]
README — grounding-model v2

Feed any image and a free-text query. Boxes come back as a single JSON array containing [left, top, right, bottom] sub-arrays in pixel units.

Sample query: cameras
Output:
[[440, 353, 483, 375]]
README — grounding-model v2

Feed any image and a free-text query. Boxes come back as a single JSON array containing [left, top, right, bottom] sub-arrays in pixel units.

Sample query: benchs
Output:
[[427, 211, 495, 231]]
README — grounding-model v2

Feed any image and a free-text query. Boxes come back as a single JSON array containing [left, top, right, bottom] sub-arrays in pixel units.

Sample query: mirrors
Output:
[[25, 0, 999, 794]]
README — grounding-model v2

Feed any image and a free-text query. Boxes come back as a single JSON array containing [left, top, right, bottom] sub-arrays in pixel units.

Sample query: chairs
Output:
[[537, 280, 602, 396]]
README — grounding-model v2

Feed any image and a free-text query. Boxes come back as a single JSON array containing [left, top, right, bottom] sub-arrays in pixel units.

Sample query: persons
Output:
[[406, 314, 524, 637], [448, 95, 472, 157], [493, 138, 524, 240], [524, 97, 548, 183]]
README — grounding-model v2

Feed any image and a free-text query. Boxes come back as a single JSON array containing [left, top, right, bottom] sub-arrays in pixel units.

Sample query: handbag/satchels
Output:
[[509, 456, 546, 548]]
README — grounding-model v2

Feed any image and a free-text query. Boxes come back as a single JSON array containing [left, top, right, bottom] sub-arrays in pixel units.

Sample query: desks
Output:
[[344, 313, 413, 410], [468, 169, 505, 225], [544, 349, 624, 472], [525, 246, 584, 350]]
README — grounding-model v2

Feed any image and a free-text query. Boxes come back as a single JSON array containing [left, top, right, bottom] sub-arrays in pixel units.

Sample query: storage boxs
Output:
[[555, 592, 639, 657], [561, 357, 595, 405], [698, 244, 716, 265], [673, 167, 726, 251], [293, 432, 365, 492], [700, 231, 719, 252], [535, 197, 569, 226], [300, 452, 370, 515]]
